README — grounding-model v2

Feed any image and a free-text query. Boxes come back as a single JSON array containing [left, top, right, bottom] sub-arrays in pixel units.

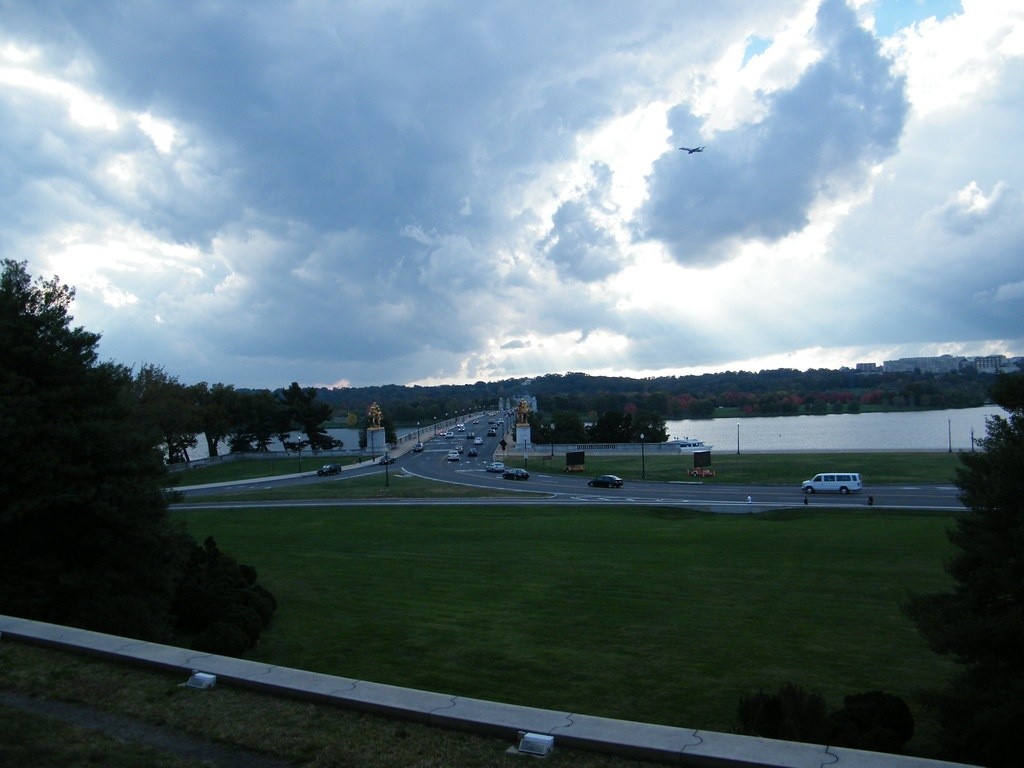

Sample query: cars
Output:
[[588, 475, 623, 488], [474, 437, 483, 445], [412, 443, 424, 453], [454, 446, 464, 453], [486, 462, 505, 473], [446, 432, 454, 439], [317, 464, 341, 476], [468, 447, 478, 456], [448, 451, 459, 461], [502, 469, 529, 480], [440, 430, 447, 436], [380, 455, 395, 464], [488, 408, 513, 416], [456, 418, 504, 439]]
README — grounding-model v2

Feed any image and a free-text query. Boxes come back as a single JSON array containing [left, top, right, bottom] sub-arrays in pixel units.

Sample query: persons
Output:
[[359, 457, 361, 463], [866, 495, 874, 506], [748, 494, 751, 504], [804, 495, 808, 505], [372, 456, 375, 462]]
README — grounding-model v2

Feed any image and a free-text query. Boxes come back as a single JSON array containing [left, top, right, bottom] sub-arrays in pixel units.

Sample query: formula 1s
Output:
[[688, 467, 716, 478]]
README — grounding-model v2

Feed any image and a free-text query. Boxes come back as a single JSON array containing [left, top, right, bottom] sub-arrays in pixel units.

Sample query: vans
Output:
[[801, 472, 862, 494]]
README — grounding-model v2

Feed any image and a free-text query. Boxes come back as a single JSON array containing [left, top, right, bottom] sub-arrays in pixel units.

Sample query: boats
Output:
[[671, 437, 713, 452]]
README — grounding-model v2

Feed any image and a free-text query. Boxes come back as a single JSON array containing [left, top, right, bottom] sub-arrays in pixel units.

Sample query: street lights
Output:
[[469, 408, 471, 418], [971, 426, 974, 452], [417, 422, 420, 443], [737, 423, 739, 454], [640, 433, 645, 479], [475, 407, 476, 416], [510, 412, 511, 426], [455, 410, 457, 422], [506, 415, 508, 433], [298, 437, 300, 472], [463, 409, 464, 421], [948, 419, 951, 452], [446, 413, 447, 430], [434, 416, 436, 435]]
